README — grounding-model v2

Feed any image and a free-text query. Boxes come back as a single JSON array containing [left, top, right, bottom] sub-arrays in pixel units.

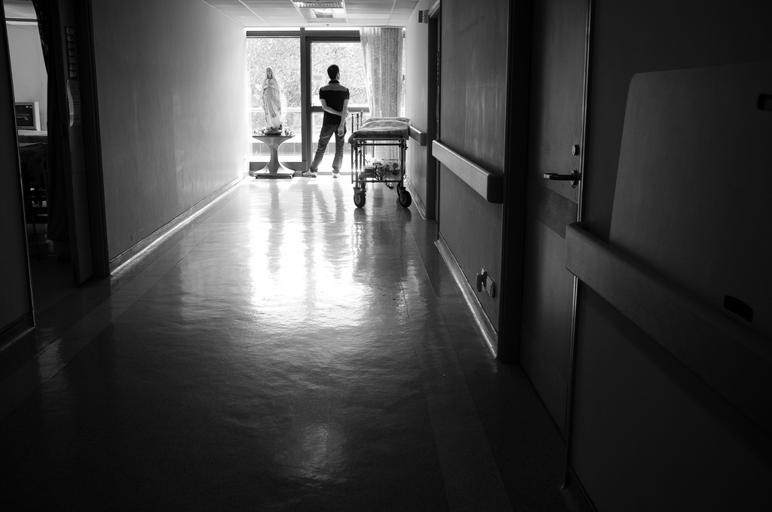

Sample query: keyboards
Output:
[[18, 129, 47, 137]]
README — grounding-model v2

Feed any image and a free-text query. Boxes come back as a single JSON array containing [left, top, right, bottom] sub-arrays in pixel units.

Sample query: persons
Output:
[[262, 65, 281, 132], [303, 64, 351, 179]]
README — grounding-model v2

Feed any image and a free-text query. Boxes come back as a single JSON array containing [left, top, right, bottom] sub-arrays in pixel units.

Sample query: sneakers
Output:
[[302, 169, 316, 177], [333, 173, 339, 178]]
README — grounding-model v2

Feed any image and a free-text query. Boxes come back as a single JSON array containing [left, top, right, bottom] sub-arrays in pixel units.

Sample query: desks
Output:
[[251, 133, 296, 177]]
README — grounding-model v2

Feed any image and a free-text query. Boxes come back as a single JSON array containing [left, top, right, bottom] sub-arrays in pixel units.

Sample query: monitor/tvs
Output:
[[15, 101, 40, 131]]
